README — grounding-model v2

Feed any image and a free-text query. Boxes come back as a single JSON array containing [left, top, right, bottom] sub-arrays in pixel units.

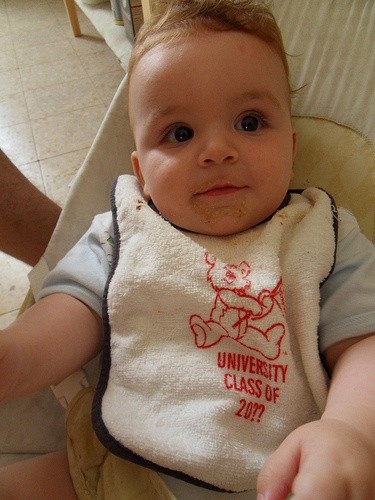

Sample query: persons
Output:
[[0, 148, 61, 266], [0, 0, 374, 500]]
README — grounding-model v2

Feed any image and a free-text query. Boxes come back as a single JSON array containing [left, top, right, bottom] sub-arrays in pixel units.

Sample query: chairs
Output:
[[0, 0, 375, 500]]
